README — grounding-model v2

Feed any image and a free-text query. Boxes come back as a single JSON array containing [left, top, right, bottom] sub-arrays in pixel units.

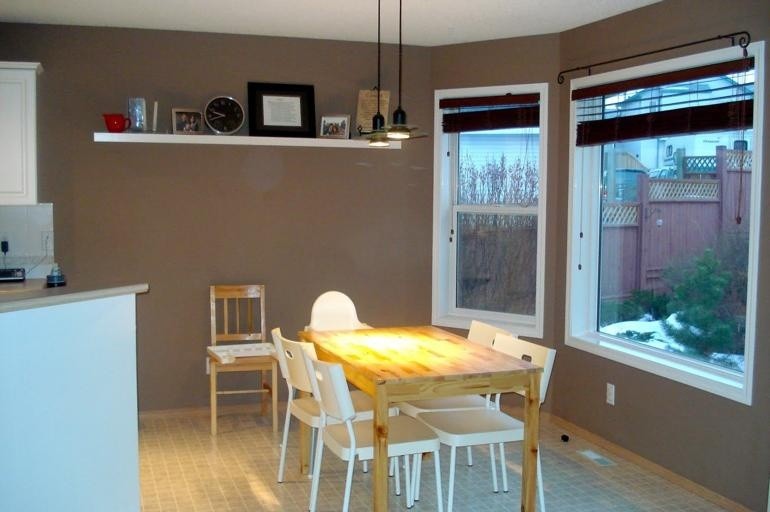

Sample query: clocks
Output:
[[204, 95, 245, 134]]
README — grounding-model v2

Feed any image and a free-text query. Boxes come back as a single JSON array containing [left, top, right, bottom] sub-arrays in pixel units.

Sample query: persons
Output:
[[324, 123, 343, 136], [177, 114, 200, 132]]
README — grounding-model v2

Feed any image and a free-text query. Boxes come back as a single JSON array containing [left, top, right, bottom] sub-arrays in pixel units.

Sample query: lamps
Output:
[[359, 0, 428, 147]]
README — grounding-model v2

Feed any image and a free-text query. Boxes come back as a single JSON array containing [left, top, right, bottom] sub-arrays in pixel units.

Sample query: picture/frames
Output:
[[171, 108, 203, 135], [247, 81, 351, 139]]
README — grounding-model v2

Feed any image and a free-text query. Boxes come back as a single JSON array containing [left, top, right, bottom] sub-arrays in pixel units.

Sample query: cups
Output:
[[102, 113, 132, 133]]
